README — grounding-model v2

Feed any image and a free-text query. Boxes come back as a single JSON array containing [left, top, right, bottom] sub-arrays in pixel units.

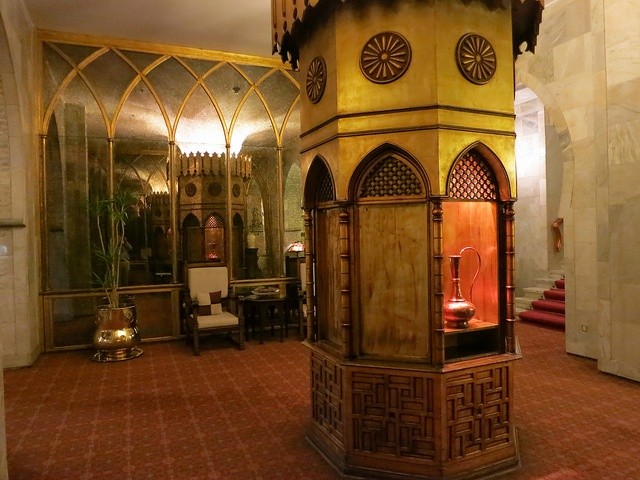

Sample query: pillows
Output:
[[196, 291, 222, 316]]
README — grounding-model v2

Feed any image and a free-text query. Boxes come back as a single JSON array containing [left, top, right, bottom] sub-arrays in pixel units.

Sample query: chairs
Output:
[[182, 263, 245, 356]]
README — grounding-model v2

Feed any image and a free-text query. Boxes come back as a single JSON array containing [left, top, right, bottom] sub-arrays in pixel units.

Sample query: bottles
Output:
[[207, 242, 220, 261]]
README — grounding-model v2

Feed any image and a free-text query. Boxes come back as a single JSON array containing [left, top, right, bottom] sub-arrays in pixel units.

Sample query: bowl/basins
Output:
[[252, 287, 280, 297]]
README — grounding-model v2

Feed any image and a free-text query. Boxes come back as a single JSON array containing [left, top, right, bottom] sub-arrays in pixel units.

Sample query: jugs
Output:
[[442, 247, 483, 330]]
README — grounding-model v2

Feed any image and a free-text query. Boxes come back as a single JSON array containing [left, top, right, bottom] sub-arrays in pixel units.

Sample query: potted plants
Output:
[[83, 178, 143, 362]]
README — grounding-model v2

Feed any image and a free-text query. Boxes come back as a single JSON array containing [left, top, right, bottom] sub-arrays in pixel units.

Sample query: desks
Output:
[[243, 296, 286, 344]]
